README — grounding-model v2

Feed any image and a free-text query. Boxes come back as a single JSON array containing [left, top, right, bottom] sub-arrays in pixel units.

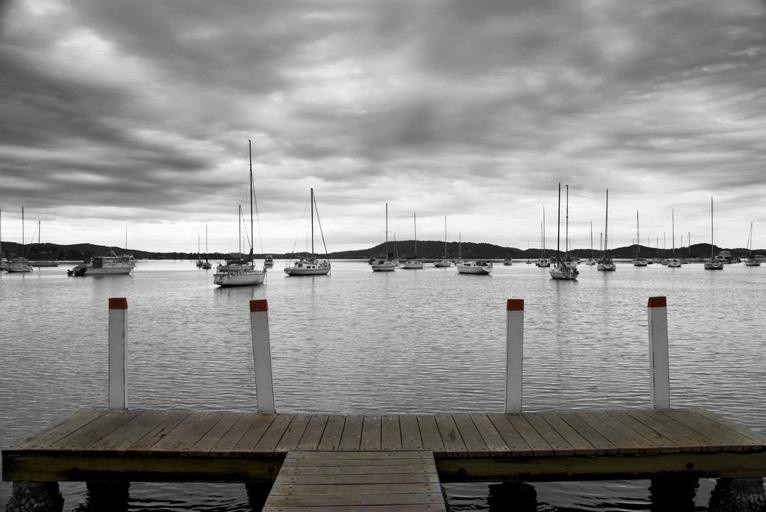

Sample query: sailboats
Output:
[[0, 205, 136, 275]]
